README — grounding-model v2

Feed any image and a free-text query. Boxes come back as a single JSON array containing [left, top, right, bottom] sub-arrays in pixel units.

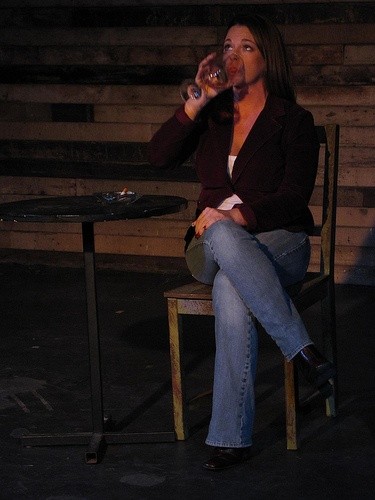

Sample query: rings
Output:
[[202, 225, 207, 229]]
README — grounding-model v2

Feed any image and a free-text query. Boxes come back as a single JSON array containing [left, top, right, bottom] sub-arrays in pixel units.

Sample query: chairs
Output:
[[164, 122, 341, 450]]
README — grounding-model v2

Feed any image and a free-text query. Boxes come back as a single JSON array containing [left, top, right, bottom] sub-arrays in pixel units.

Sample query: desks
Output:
[[0, 192, 189, 464]]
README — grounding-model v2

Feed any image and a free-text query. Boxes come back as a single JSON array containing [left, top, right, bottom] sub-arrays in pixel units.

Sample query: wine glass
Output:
[[178, 50, 245, 107]]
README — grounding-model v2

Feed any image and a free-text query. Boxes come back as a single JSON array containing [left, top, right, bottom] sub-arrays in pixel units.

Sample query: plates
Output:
[[95, 191, 143, 204]]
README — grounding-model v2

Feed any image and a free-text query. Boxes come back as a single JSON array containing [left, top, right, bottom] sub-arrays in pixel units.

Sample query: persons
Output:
[[136, 15, 338, 471]]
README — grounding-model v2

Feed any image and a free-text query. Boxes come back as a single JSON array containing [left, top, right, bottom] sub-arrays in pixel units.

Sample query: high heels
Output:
[[293, 345, 336, 398], [200, 445, 245, 470]]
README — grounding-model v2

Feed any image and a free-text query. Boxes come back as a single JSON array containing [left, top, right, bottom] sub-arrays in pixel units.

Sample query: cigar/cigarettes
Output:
[[120, 188, 127, 196]]
